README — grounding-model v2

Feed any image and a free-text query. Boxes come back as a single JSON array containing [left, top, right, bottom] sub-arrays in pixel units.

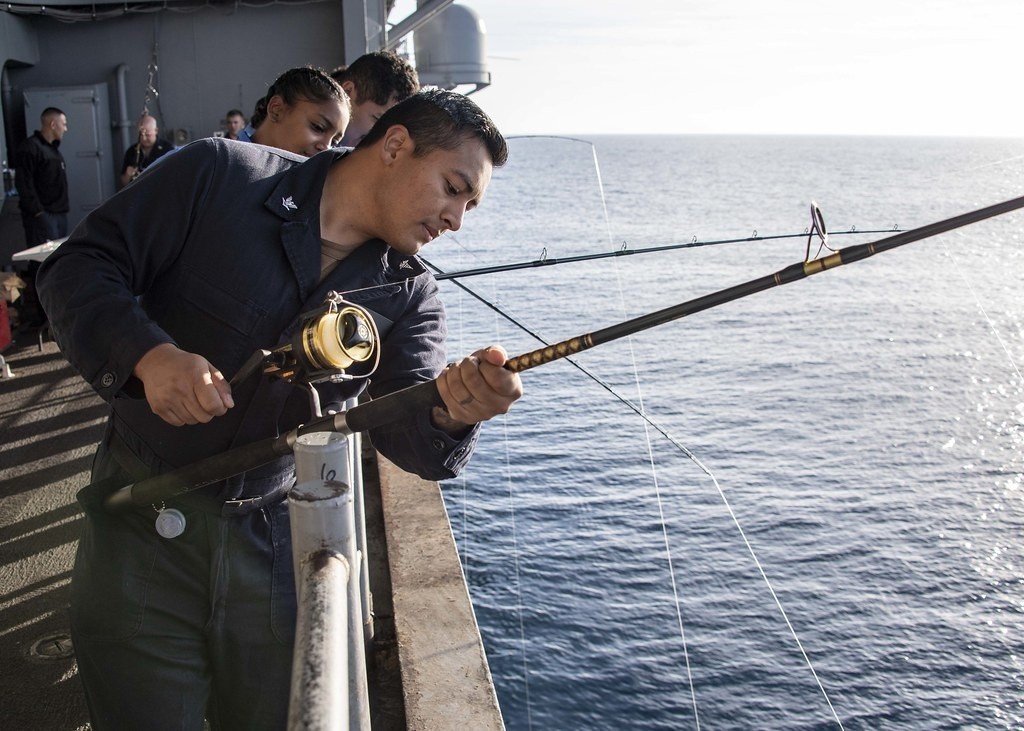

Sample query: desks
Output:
[[9, 237, 69, 352]]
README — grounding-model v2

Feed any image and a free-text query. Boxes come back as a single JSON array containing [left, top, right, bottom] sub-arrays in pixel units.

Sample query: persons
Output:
[[35, 88, 522, 731], [224, 50, 420, 158], [122, 116, 175, 187], [14, 107, 70, 327]]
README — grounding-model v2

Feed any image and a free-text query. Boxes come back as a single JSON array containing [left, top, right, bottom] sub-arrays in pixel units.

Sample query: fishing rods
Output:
[[337, 227, 907, 296], [75, 193, 1024, 531], [415, 254, 842, 730], [501, 134, 701, 730]]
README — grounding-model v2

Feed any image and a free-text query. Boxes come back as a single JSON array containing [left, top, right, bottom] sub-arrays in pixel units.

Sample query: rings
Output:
[[459, 394, 474, 405]]
